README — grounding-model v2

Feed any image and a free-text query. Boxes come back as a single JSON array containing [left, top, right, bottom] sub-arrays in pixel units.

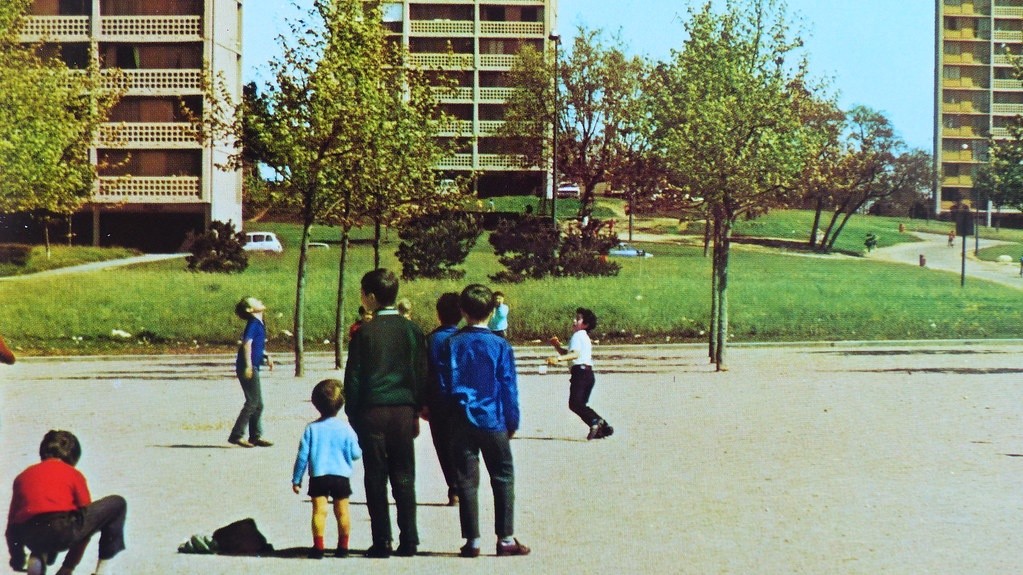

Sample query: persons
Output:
[[426, 291, 463, 506], [477, 197, 494, 212], [397, 297, 412, 317], [348, 306, 372, 341], [228, 296, 275, 447], [343, 268, 429, 558], [488, 292, 509, 338], [865, 231, 877, 253], [6, 430, 127, 575], [546, 306, 614, 440], [947, 230, 955, 246], [291, 379, 362, 560], [435, 284, 531, 558]]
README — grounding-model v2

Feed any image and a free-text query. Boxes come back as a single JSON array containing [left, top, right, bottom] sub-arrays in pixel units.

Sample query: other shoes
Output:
[[308, 547, 323, 557], [249, 437, 273, 446], [334, 548, 349, 558], [450, 496, 458, 505], [397, 542, 417, 555], [460, 544, 479, 556], [228, 436, 253, 447], [587, 418, 608, 440], [497, 537, 530, 556], [367, 540, 392, 554], [593, 424, 613, 438], [27, 552, 45, 575]]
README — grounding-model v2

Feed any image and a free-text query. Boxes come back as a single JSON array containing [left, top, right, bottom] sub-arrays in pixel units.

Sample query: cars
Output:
[[241, 231, 282, 256], [557, 180, 580, 198], [655, 188, 704, 203], [609, 243, 653, 257], [432, 180, 459, 194], [309, 244, 329, 249], [604, 186, 628, 199]]
